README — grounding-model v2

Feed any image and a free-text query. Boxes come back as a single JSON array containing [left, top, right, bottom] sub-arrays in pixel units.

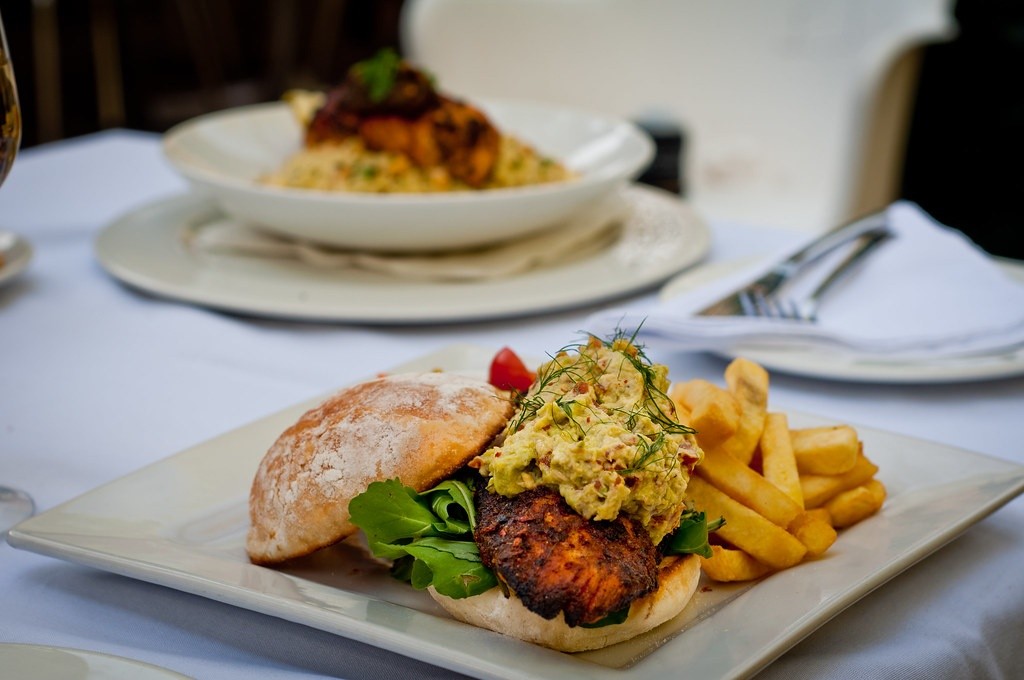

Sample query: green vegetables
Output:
[[348, 49, 404, 102]]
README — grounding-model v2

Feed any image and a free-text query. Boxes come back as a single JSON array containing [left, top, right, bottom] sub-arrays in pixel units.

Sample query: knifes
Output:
[[696, 226, 858, 315]]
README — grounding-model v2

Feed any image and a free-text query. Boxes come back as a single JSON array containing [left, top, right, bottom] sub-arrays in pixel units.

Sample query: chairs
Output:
[[860, 33, 1024, 263]]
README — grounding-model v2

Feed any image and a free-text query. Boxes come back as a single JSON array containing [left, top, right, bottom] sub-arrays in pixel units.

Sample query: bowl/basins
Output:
[[168, 108, 657, 250]]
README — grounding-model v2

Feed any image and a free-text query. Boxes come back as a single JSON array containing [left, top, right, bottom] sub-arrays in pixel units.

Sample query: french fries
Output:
[[662, 356, 887, 583]]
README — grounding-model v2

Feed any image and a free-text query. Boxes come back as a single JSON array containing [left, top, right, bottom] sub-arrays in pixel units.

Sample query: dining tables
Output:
[[0, 129, 1024, 680]]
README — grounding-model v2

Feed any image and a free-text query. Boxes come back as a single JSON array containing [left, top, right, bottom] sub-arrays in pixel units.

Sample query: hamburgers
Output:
[[245, 320, 725, 655]]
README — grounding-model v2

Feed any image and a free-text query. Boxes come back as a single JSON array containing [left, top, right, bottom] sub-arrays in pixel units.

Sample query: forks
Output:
[[740, 227, 894, 317]]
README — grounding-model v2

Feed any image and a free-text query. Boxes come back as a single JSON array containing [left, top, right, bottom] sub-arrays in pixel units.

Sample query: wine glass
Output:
[[0, 19, 33, 282], [0, 488, 36, 536]]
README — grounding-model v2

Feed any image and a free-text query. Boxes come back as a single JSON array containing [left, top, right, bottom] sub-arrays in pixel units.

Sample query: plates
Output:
[[0, 643, 193, 680], [661, 262, 1024, 382], [98, 186, 713, 322], [6, 341, 1024, 680]]
